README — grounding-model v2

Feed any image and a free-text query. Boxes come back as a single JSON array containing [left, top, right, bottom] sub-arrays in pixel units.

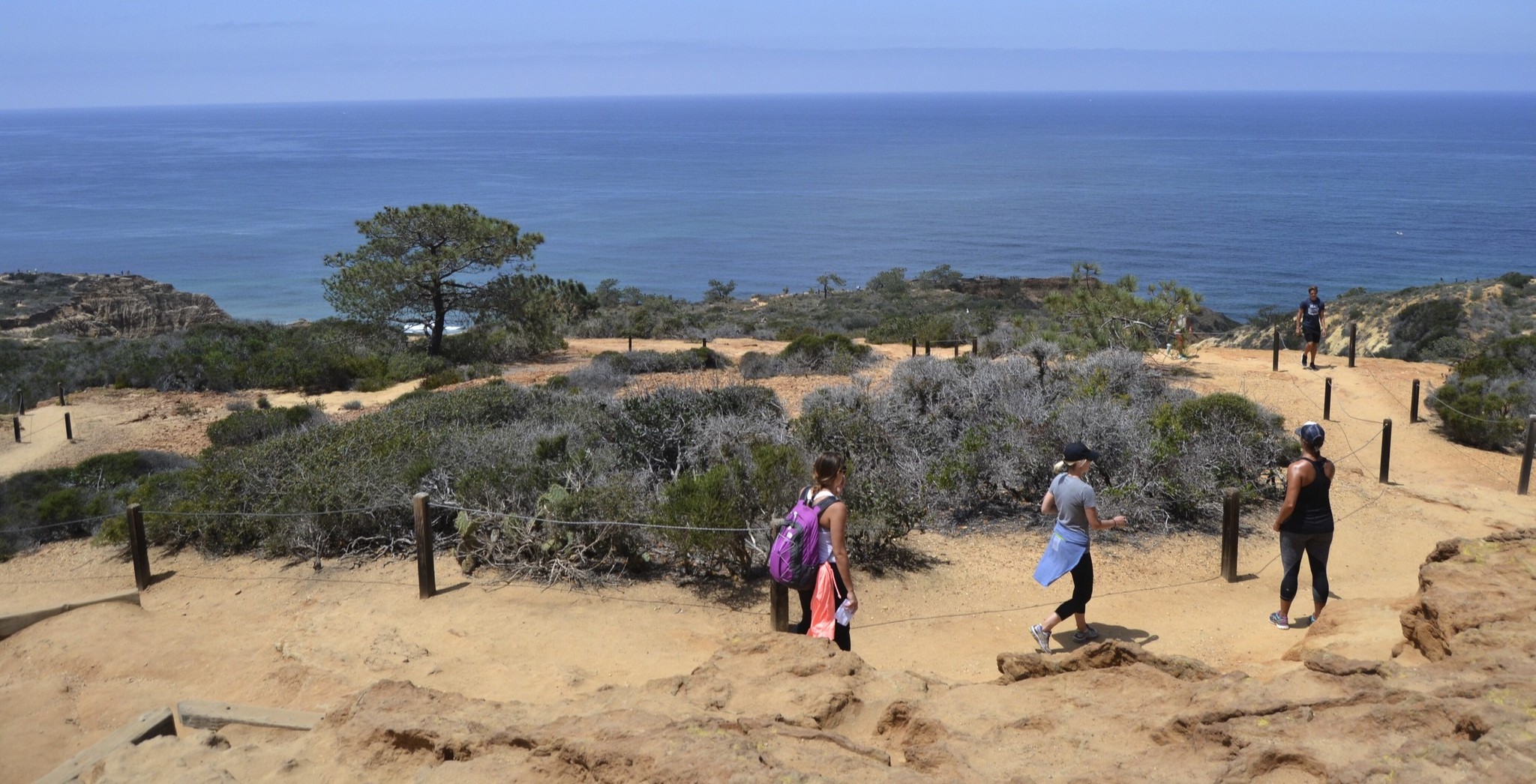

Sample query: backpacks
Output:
[[769, 485, 843, 591]]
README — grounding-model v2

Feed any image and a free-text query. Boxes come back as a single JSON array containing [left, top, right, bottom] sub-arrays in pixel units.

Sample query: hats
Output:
[[1294, 420, 1325, 447], [1063, 442, 1099, 462]]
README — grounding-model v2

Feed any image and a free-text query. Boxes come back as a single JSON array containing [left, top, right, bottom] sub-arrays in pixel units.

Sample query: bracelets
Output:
[[1112, 519, 1116, 528]]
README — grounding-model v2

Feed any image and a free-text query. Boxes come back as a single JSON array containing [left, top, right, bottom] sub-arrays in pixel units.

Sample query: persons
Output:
[[1296, 286, 1327, 370], [114, 272, 116, 276], [1168, 297, 1193, 361], [1269, 420, 1335, 630], [795, 453, 858, 653], [1027, 441, 1128, 653], [104, 273, 111, 277], [121, 270, 123, 275], [97, 273, 98, 279], [27, 270, 30, 272], [18, 269, 19, 273], [35, 267, 37, 274], [127, 269, 130, 275]]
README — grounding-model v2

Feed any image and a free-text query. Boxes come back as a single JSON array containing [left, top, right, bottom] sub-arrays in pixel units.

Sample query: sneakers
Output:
[[1307, 612, 1315, 626], [1027, 623, 1052, 655], [1309, 362, 1317, 371], [1269, 610, 1289, 630], [1301, 353, 1308, 367], [1072, 623, 1099, 643]]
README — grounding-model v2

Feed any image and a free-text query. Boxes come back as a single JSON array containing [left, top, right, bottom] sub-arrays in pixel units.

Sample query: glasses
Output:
[[840, 466, 847, 475]]
